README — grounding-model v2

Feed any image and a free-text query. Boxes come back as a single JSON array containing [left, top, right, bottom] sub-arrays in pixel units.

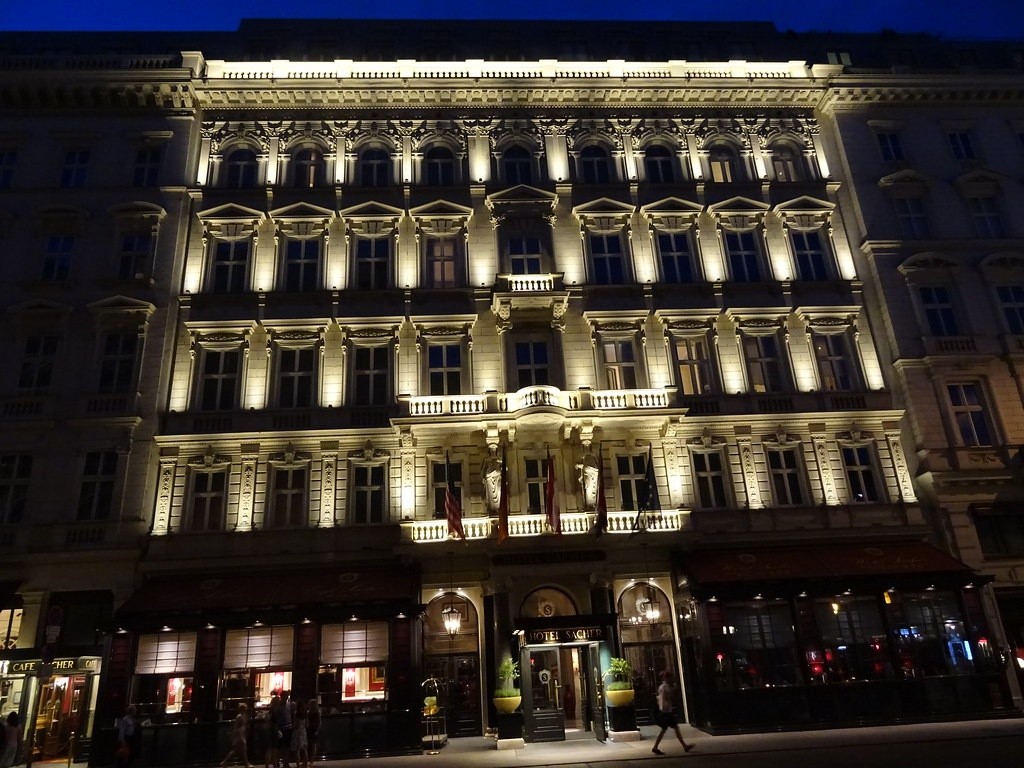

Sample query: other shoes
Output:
[[685, 744, 695, 752], [653, 748, 664, 755]]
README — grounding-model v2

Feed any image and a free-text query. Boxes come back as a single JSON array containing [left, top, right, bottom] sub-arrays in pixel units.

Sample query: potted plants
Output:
[[492, 657, 522, 713], [601, 656, 635, 707]]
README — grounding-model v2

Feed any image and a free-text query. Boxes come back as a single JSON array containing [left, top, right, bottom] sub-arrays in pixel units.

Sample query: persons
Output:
[[633, 670, 659, 722], [118, 706, 136, 766], [265, 692, 322, 768], [652, 670, 694, 755], [221, 703, 255, 768], [0, 712, 23, 768]]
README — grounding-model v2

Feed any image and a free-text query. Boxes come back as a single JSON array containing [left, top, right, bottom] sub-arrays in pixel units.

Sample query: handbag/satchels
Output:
[[112, 742, 128, 760], [17, 727, 24, 752]]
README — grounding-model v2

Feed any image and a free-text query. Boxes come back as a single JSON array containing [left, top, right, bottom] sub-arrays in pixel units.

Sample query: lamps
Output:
[[441, 552, 461, 640], [638, 542, 660, 630]]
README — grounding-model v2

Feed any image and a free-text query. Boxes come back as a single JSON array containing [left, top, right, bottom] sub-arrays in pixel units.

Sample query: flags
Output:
[[643, 464, 661, 513], [593, 452, 608, 541], [547, 457, 555, 525], [497, 452, 508, 546], [445, 452, 465, 538]]
